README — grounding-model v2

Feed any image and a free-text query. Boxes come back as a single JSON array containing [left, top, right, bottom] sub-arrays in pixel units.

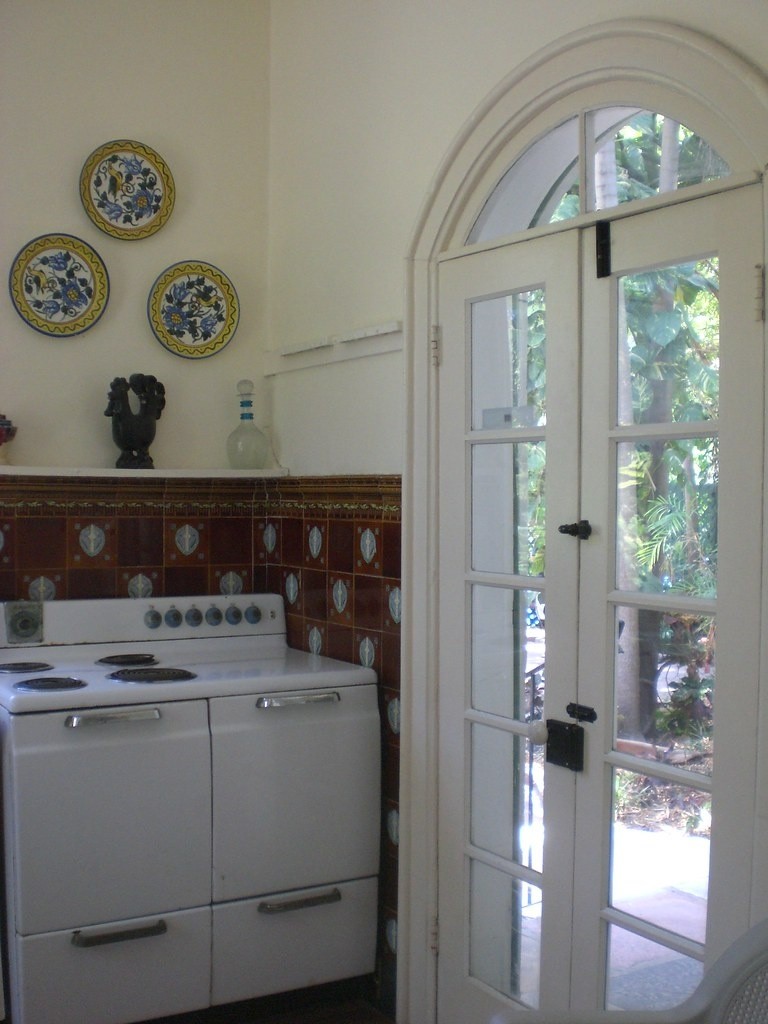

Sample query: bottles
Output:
[[226, 379, 268, 470]]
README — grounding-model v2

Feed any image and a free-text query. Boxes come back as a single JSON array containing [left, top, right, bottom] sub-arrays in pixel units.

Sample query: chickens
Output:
[[103, 373, 165, 469]]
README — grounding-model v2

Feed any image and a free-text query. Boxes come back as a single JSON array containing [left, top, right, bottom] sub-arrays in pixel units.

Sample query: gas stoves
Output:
[[0, 594, 377, 716]]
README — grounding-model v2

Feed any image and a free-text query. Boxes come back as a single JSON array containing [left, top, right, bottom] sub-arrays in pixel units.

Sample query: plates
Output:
[[8, 233, 110, 337], [79, 140, 176, 241], [147, 260, 239, 359]]
